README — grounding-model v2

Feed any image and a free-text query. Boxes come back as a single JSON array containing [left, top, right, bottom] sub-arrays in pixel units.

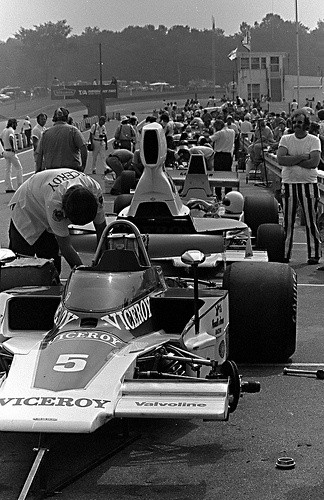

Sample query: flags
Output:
[[241, 30, 251, 51], [226, 47, 237, 61]]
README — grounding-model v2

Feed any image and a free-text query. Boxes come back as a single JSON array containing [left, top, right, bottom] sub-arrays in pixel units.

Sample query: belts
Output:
[[94, 139, 104, 141], [109, 154, 121, 161]]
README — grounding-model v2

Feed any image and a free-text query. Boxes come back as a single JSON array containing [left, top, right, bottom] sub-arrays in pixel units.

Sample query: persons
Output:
[[6, 168, 109, 270], [110, 75, 117, 85], [51, 76, 60, 85], [104, 148, 134, 175], [20, 115, 33, 149], [89, 116, 112, 174], [206, 119, 235, 200], [35, 108, 89, 173], [277, 112, 322, 265], [92, 78, 98, 86], [114, 93, 324, 173], [31, 113, 48, 170], [0, 118, 23, 193]]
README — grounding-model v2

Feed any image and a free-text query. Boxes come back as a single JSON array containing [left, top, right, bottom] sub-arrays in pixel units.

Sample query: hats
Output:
[[65, 188, 97, 226], [8, 119, 21, 124], [120, 116, 129, 122], [54, 107, 69, 117]]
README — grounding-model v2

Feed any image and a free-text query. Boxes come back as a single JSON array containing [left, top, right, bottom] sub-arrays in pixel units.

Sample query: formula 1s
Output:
[[66, 120, 288, 289], [0, 218, 299, 452]]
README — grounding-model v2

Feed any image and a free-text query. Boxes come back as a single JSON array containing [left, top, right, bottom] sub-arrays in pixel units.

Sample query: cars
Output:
[[0, 78, 171, 104]]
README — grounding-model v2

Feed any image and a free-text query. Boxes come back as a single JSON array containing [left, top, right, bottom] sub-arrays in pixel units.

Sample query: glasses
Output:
[[292, 120, 306, 124]]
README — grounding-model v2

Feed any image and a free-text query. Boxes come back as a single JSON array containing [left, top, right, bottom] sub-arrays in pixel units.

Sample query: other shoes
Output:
[[5, 189, 16, 193], [284, 258, 289, 263], [92, 170, 96, 174], [308, 257, 320, 264]]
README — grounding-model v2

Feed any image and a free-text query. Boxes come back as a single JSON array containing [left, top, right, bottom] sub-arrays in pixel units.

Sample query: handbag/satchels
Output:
[[87, 143, 93, 151], [113, 140, 119, 149]]
